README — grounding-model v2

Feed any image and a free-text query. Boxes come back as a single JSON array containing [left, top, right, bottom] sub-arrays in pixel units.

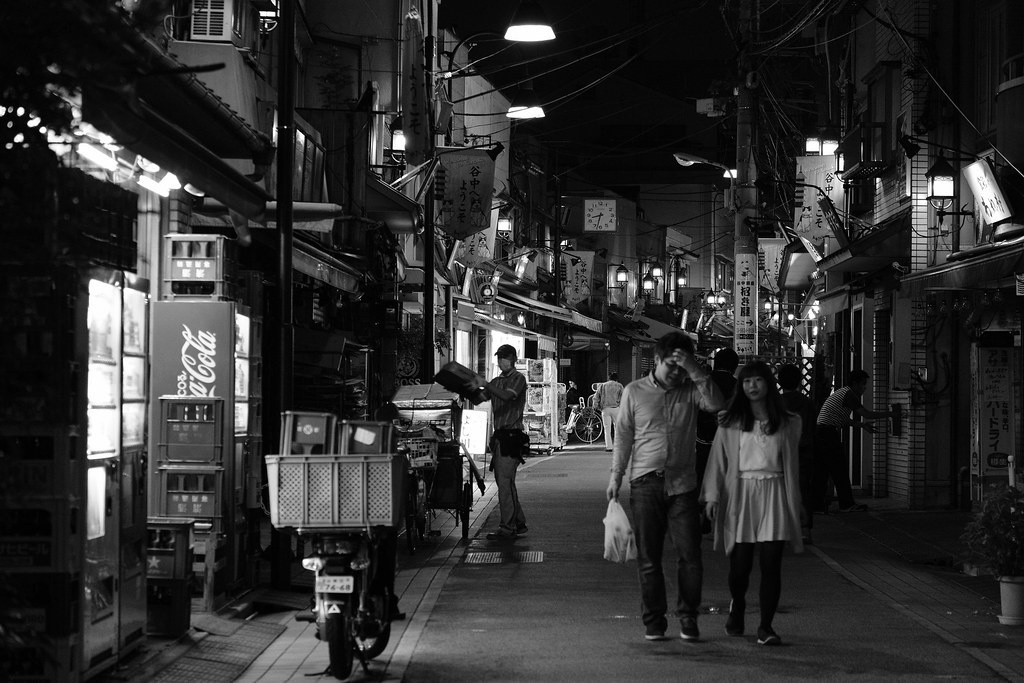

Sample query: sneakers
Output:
[[646, 622, 666, 640], [757, 626, 781, 644], [680, 618, 702, 639], [517, 526, 527, 533], [725, 608, 747, 637], [487, 529, 517, 539], [846, 504, 869, 514]]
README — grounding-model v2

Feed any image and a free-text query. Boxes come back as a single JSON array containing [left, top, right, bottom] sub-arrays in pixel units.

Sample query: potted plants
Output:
[[954, 481, 1024, 618]]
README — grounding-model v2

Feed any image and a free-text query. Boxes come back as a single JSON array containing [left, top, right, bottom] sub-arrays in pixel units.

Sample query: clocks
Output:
[[581, 197, 620, 234]]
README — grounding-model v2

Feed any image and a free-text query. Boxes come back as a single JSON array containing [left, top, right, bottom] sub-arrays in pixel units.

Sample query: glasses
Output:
[[662, 359, 687, 374]]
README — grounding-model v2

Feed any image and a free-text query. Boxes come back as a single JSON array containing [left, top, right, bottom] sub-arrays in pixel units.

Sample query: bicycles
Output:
[[562, 397, 604, 445]]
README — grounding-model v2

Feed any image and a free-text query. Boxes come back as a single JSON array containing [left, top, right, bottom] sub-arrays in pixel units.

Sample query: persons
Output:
[[818, 368, 907, 514], [698, 360, 803, 646], [468, 345, 528, 540], [699, 347, 739, 534], [816, 354, 840, 412], [778, 363, 816, 547], [592, 373, 625, 452], [566, 374, 578, 445], [607, 330, 730, 640]]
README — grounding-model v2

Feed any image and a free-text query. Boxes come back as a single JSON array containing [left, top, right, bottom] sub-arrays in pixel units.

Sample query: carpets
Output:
[[189, 612, 243, 637]]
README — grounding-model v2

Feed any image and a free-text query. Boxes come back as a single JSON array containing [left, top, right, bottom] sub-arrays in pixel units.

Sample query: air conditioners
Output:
[[191, 0, 237, 45]]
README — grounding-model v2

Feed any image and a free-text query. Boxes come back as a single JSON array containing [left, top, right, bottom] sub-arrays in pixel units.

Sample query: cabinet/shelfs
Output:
[[515, 358, 569, 456]]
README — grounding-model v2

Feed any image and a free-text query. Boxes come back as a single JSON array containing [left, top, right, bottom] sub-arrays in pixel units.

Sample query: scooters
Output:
[[295, 526, 406, 681]]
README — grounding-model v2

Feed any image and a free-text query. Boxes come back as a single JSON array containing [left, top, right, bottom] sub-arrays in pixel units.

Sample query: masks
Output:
[[498, 357, 513, 371]]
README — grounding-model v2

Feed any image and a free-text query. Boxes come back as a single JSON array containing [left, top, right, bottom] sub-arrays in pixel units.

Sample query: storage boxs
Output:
[[265, 410, 405, 528], [164, 232, 236, 301], [435, 361, 487, 405], [157, 393, 229, 613]]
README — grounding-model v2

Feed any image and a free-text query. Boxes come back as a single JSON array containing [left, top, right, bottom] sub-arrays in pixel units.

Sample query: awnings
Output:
[[497, 290, 603, 333], [613, 311, 698, 344], [89, 82, 274, 229]]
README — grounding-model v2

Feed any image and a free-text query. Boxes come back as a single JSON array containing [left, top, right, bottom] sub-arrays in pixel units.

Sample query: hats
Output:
[[494, 345, 518, 356]]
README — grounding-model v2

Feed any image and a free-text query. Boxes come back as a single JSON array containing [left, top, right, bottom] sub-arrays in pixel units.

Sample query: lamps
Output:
[[504, 0, 556, 42], [495, 214, 512, 242], [497, 248, 538, 263], [608, 260, 628, 293], [370, 113, 407, 177], [585, 247, 609, 259], [636, 261, 687, 300], [923, 149, 976, 226], [899, 134, 980, 160], [702, 287, 726, 309], [531, 238, 582, 266], [505, 78, 546, 119], [927, 275, 1004, 316]]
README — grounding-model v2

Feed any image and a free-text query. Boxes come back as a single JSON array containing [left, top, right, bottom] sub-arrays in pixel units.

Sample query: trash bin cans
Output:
[[143, 519, 196, 638]]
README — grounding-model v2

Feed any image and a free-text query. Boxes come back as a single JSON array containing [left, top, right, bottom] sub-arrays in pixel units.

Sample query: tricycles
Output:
[[392, 419, 474, 556]]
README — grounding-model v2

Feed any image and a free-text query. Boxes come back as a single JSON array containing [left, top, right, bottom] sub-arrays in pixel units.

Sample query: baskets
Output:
[[398, 438, 440, 468]]
